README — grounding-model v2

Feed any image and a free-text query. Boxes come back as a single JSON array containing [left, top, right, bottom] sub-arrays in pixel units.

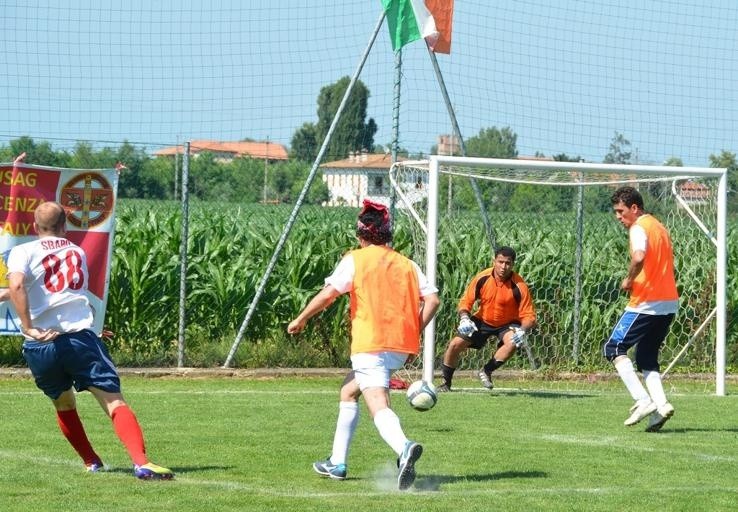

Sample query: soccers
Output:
[[408, 381, 438, 411]]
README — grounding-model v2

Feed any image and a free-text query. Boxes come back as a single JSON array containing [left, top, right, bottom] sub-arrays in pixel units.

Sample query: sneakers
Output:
[[86, 463, 108, 471], [478, 368, 494, 388], [396, 441, 423, 490], [436, 384, 450, 392], [624, 402, 657, 426], [313, 459, 347, 481], [645, 400, 674, 432], [133, 463, 175, 480]]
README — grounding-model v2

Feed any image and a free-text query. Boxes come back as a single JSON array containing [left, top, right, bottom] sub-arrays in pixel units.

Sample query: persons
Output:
[[287, 199, 441, 491], [0, 201, 175, 481], [603, 186, 680, 433], [434, 246, 537, 393]]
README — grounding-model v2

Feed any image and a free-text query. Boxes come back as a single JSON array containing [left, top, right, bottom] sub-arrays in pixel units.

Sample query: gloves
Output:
[[509, 326, 527, 347], [458, 315, 478, 337]]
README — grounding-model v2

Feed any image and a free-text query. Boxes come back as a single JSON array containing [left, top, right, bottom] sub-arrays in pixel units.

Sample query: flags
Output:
[[380, 0, 455, 54]]
